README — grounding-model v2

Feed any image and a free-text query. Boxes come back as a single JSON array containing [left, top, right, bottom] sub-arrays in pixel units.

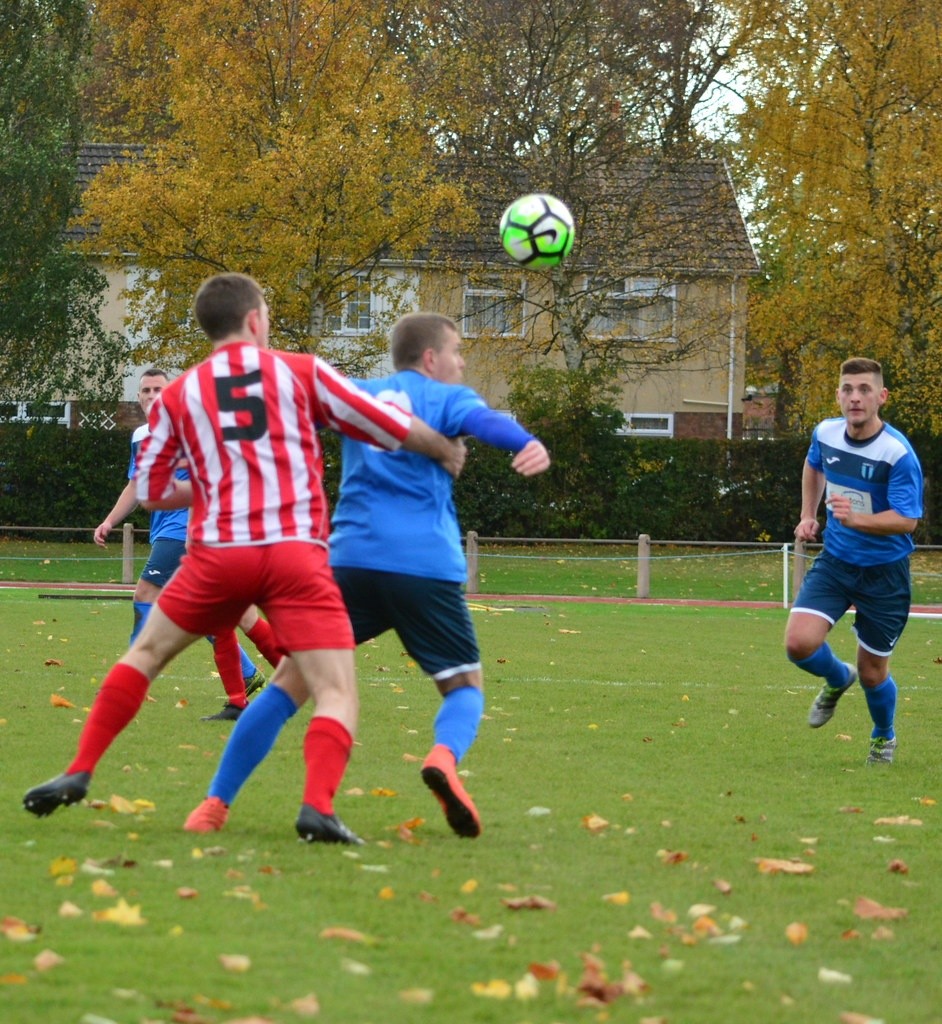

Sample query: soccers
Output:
[[499, 192, 577, 272]]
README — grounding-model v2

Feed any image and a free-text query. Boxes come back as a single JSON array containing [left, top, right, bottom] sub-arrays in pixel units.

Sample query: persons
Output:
[[22, 273, 465, 846], [93, 368, 284, 722], [783, 359, 925, 762], [184, 312, 550, 839]]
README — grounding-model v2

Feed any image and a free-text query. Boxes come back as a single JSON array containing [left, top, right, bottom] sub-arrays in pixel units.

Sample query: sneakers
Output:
[[420, 745, 481, 838], [184, 796, 230, 833], [243, 668, 266, 697], [296, 803, 365, 845], [866, 736, 897, 765], [22, 771, 90, 818], [199, 700, 250, 721], [807, 662, 857, 728]]
